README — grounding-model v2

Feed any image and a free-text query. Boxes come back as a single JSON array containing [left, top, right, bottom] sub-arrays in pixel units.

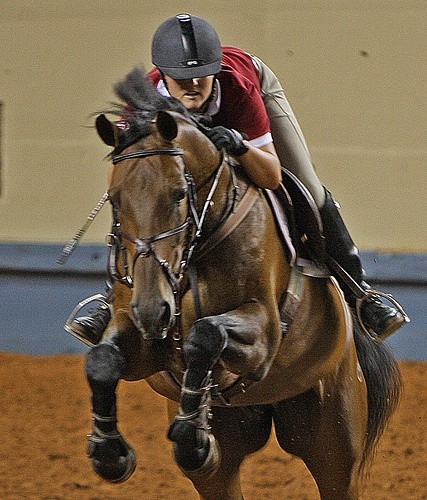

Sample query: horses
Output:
[[83, 68, 405, 500]]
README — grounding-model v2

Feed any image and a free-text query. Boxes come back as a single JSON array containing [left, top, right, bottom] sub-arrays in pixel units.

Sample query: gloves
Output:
[[207, 126, 250, 154]]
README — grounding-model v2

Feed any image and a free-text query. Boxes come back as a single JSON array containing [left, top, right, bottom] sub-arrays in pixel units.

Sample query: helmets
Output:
[[150, 13, 222, 80]]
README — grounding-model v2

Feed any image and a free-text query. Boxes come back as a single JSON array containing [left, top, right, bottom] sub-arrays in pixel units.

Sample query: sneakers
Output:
[[69, 306, 110, 344], [360, 300, 405, 337]]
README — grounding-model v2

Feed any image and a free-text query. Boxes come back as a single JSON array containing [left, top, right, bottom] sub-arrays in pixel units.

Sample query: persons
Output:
[[63, 12, 413, 350]]
